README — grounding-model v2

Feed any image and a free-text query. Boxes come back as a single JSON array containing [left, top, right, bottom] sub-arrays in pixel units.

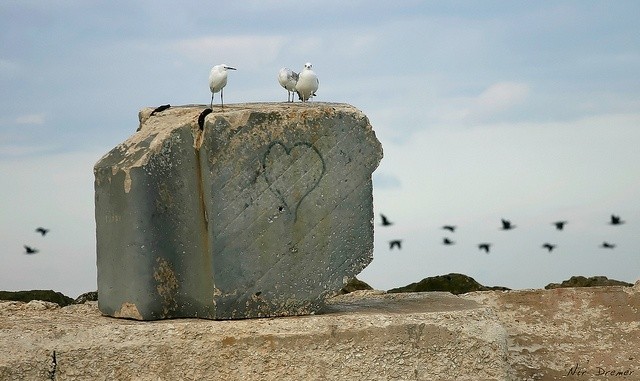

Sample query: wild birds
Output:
[[23, 227, 49, 255], [380, 213, 392, 227], [552, 221, 567, 230], [541, 243, 556, 252], [209, 63, 237, 108], [477, 243, 491, 253], [389, 240, 402, 249], [441, 224, 456, 233], [499, 219, 515, 231], [443, 238, 453, 245], [608, 214, 625, 226], [601, 241, 615, 249], [279, 61, 320, 104]]
